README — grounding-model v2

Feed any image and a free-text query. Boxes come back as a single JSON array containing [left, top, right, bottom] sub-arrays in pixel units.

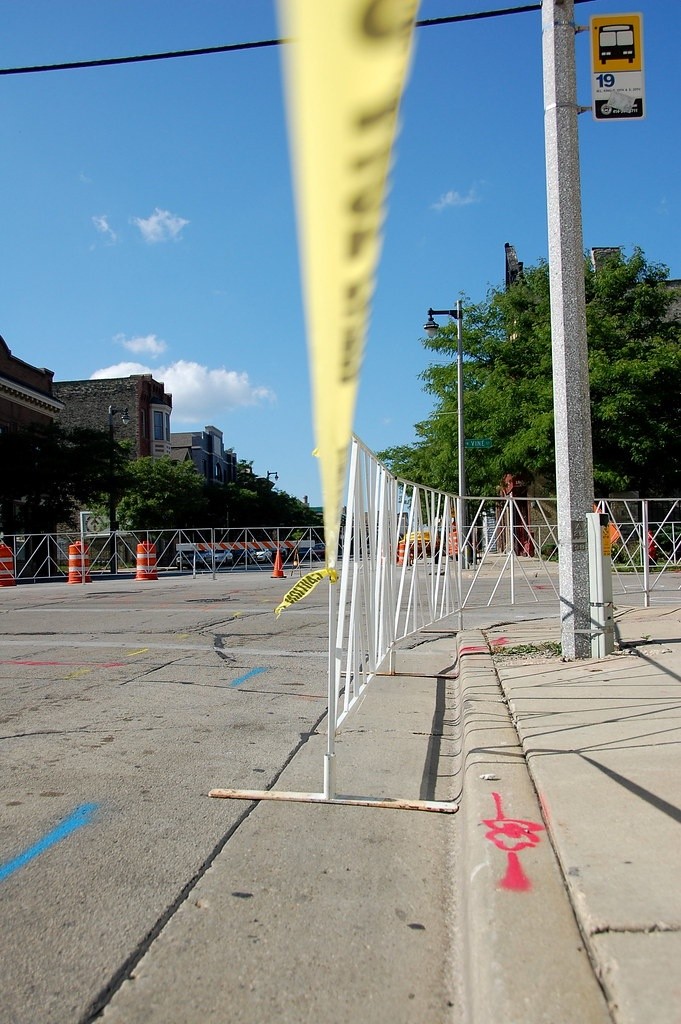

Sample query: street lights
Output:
[[423, 299, 469, 569], [267, 471, 279, 481], [109, 405, 131, 573]]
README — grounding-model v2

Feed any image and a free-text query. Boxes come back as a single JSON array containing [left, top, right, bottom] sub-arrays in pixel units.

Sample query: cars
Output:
[[176, 550, 217, 570], [233, 548, 256, 565], [213, 549, 233, 567], [299, 543, 325, 561], [253, 549, 272, 563]]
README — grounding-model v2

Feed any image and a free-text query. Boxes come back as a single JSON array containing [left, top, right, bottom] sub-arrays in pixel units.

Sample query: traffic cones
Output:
[[270, 549, 287, 578]]
